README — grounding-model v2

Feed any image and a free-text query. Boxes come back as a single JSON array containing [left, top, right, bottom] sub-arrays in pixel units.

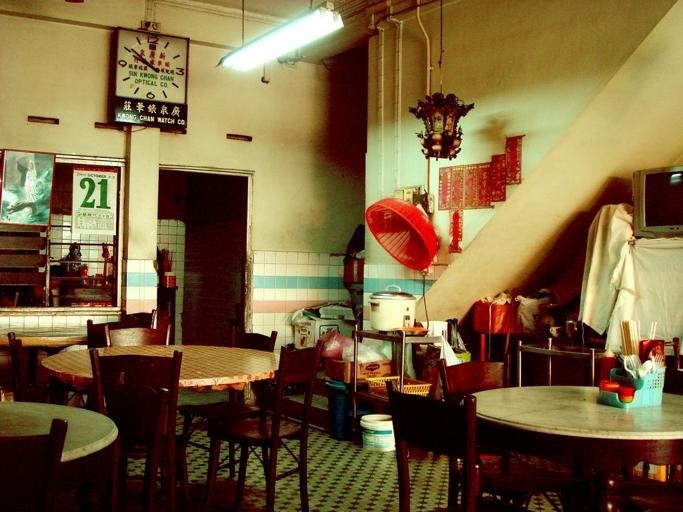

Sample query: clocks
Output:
[[100, 24, 192, 132]]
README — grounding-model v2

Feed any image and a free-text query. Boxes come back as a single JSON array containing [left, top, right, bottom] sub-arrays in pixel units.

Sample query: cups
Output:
[[567, 320, 580, 339], [549, 326, 563, 337]]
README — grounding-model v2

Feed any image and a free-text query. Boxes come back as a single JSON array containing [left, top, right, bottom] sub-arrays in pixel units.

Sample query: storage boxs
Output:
[[291, 308, 351, 350]]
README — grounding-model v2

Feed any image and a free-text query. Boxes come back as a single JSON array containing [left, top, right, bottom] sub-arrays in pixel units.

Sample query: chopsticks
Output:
[[619, 318, 659, 356]]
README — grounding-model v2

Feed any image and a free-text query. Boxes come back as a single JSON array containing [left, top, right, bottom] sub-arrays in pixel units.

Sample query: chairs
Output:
[[385, 335, 682, 512], [1, 303, 332, 512]]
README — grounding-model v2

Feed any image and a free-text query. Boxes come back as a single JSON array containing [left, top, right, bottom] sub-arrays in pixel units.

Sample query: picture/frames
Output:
[[0, 148, 54, 230]]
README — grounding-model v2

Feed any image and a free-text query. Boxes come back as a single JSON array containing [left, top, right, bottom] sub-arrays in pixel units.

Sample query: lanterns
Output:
[[408, 92, 475, 161]]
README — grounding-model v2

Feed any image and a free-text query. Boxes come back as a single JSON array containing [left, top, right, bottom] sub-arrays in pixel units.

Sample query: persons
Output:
[[25, 160, 36, 200]]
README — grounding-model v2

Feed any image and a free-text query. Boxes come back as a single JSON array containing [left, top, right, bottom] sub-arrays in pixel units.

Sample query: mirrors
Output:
[[0, 146, 123, 317]]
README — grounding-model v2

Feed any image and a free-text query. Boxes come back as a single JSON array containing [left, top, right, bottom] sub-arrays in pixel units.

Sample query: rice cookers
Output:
[[368, 285, 418, 334]]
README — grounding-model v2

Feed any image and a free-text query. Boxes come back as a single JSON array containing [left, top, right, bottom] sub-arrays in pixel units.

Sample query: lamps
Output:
[[407, 1, 478, 160], [215, 0, 349, 74]]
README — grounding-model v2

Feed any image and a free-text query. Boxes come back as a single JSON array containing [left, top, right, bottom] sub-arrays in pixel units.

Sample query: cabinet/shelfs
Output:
[[350, 325, 442, 443]]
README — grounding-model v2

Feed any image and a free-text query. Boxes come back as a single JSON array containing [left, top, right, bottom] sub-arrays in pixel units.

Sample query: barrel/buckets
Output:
[[361, 414, 396, 453], [324, 378, 348, 441]]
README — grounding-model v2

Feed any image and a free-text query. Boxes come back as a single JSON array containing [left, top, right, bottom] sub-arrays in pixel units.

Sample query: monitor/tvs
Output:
[[631, 165, 683, 239]]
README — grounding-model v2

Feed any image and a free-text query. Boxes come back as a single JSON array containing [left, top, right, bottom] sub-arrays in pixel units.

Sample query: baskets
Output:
[[366, 197, 439, 272], [364, 374, 433, 404]]
